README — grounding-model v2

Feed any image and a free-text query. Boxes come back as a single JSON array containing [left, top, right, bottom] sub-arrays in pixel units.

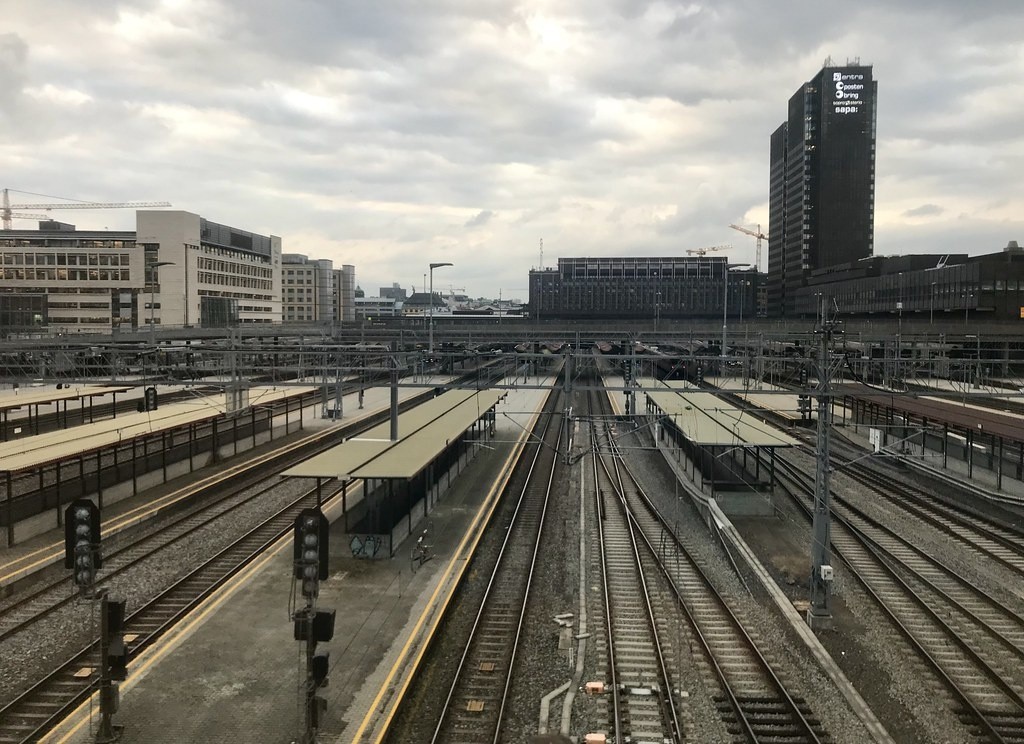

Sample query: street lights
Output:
[[429, 263, 455, 350], [147, 261, 176, 344], [896, 302, 902, 378], [721, 263, 750, 378], [965, 334, 981, 390], [655, 289, 661, 331]]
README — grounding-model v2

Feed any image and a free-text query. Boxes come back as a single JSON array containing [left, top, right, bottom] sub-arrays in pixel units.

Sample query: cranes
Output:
[[0, 187, 172, 230], [728, 223, 769, 272], [685, 244, 733, 257]]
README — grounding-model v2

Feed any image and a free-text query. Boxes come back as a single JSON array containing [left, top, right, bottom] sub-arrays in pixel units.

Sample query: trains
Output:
[[198, 343, 390, 351]]
